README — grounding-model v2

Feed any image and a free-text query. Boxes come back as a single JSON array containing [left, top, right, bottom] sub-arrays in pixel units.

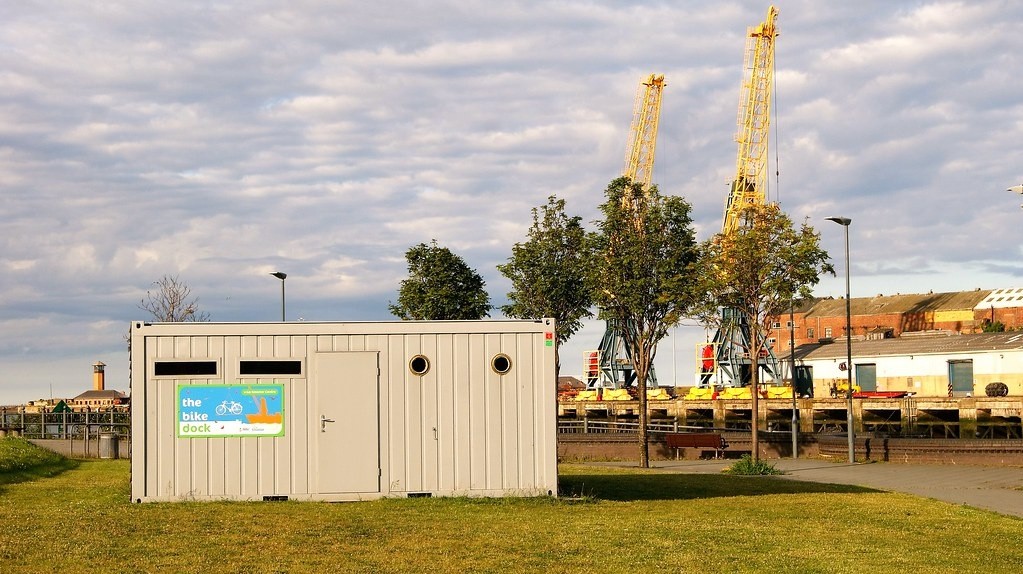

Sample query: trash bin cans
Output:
[[99, 432, 119, 459]]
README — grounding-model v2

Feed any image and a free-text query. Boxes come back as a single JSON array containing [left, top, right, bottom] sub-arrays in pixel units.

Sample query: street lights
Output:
[[824, 216, 855, 463], [773, 283, 799, 459], [268, 271, 288, 322]]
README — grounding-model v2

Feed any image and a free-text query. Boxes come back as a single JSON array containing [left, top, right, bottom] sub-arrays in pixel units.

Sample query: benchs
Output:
[[666, 433, 729, 461]]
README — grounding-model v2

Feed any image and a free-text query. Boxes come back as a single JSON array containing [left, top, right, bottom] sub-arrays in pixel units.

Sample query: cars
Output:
[[829, 379, 862, 399]]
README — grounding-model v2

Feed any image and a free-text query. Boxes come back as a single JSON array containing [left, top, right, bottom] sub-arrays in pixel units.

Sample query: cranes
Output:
[[577, 71, 670, 390], [694, 3, 787, 388]]
